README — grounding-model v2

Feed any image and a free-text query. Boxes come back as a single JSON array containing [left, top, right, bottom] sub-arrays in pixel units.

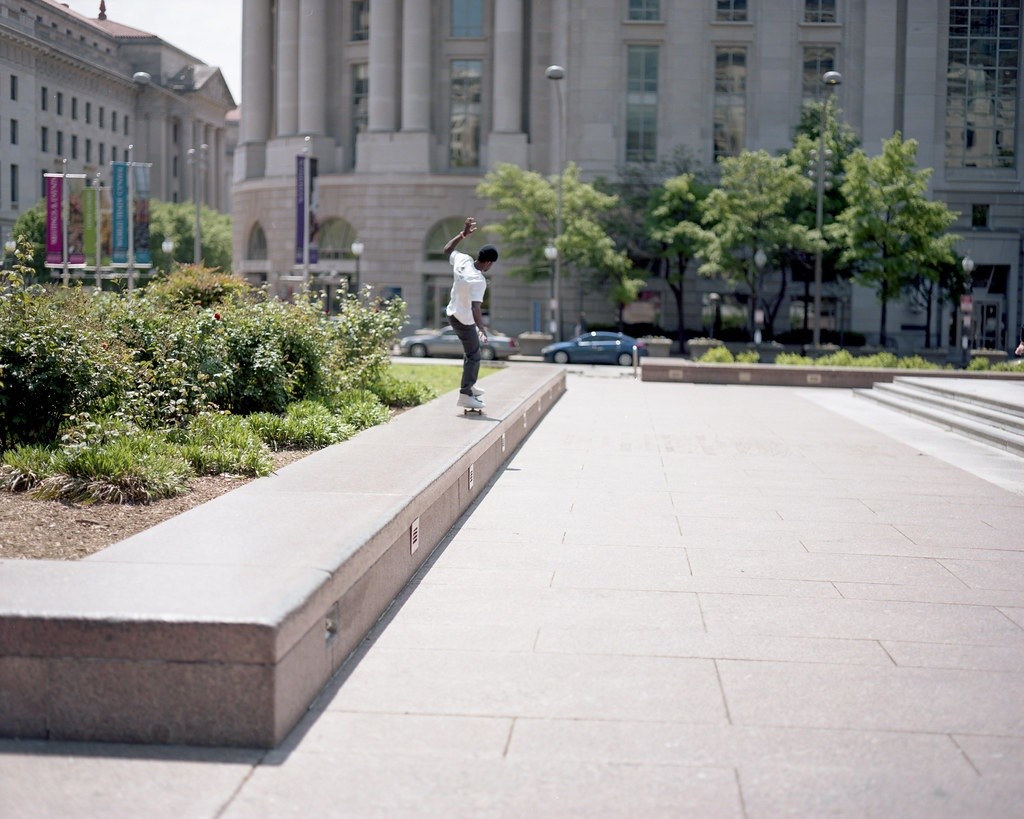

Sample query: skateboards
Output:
[[463, 395, 482, 416]]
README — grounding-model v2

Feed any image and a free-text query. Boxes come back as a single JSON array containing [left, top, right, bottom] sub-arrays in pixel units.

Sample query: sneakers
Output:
[[457, 394, 486, 408], [471, 385, 485, 396]]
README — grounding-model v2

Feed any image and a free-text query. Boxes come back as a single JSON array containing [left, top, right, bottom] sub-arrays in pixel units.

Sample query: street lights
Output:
[[543, 65, 562, 328], [751, 248, 768, 347], [960, 252, 975, 370], [160, 236, 175, 277], [351, 239, 364, 300], [543, 243, 558, 341], [130, 71, 201, 266], [5, 236, 17, 300], [812, 70, 842, 355], [187, 143, 209, 262]]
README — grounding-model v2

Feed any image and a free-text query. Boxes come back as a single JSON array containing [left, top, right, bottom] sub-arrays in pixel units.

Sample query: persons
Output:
[[443, 217, 498, 408], [1014, 323, 1024, 355]]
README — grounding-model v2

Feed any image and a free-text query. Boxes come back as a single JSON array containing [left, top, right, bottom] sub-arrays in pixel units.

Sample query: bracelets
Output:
[[460, 232, 465, 239]]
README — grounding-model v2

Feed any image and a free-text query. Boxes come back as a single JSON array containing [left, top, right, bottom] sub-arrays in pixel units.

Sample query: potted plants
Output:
[[757, 342, 783, 363], [519, 330, 553, 356], [688, 338, 723, 360], [640, 336, 673, 356]]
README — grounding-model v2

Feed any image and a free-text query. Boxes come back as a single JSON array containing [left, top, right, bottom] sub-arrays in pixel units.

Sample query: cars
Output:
[[398, 323, 519, 360], [540, 331, 644, 366]]
